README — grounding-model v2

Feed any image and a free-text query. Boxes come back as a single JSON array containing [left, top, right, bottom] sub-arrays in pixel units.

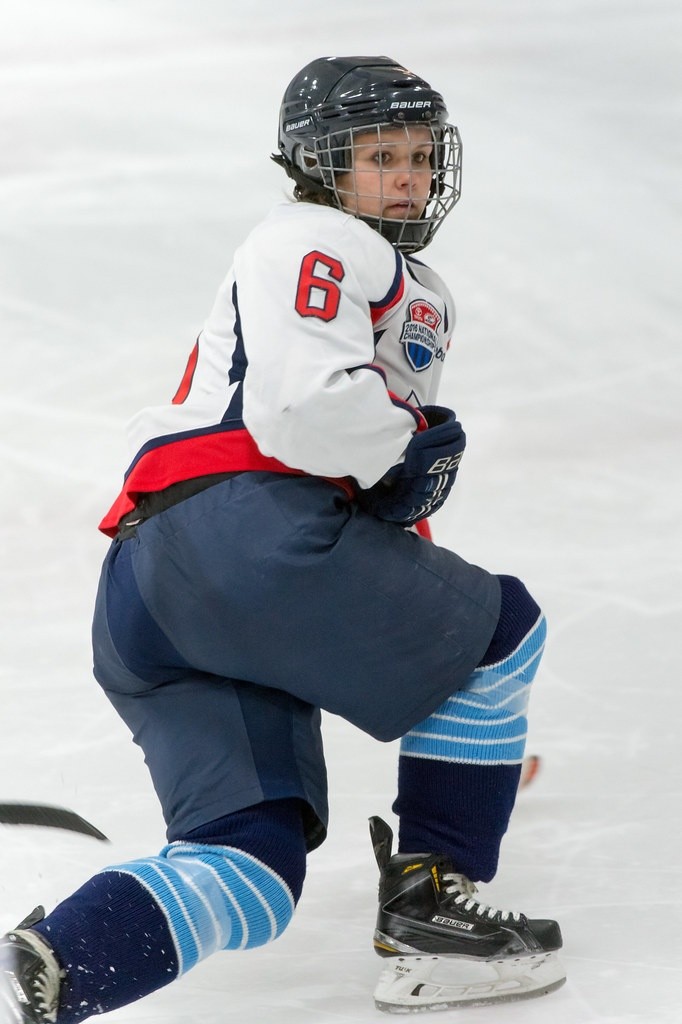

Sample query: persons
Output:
[[0, 59, 562, 1023]]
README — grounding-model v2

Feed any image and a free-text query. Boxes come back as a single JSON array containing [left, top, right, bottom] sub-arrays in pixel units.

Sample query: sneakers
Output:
[[0, 905, 58, 1024], [370, 818, 569, 1006]]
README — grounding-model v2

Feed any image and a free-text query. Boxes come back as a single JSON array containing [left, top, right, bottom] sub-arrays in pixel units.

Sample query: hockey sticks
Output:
[[1, 803, 111, 843], [415, 517, 540, 790]]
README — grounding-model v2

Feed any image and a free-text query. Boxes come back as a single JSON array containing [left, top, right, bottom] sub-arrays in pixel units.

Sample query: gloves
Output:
[[361, 404, 467, 529]]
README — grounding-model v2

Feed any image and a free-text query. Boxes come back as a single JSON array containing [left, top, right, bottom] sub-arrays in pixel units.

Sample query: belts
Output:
[[113, 468, 245, 533]]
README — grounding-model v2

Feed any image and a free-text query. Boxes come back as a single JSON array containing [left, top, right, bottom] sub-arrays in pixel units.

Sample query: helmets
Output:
[[269, 57, 463, 252]]
